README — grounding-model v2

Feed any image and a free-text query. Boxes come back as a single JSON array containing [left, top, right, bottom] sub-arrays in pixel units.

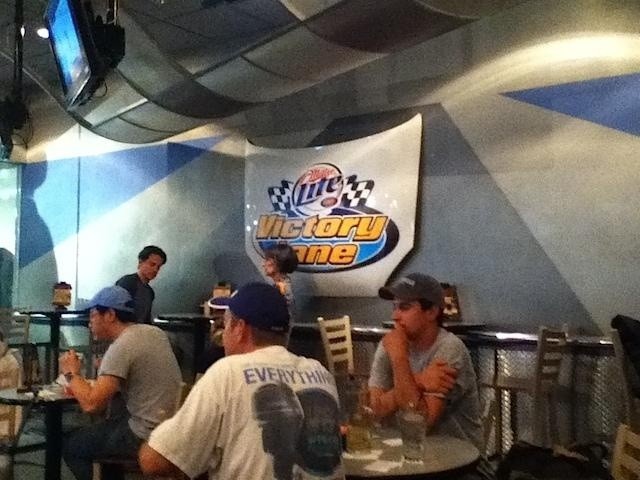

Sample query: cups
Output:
[[30, 383, 73, 399], [344, 415, 375, 455], [397, 410, 427, 465]]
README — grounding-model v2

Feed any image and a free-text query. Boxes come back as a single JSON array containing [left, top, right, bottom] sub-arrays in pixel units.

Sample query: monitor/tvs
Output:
[[42, 0, 127, 111], [0, 100, 28, 161]]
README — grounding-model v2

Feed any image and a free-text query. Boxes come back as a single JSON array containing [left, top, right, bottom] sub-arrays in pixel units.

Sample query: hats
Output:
[[75, 286, 136, 314], [207, 282, 289, 331], [379, 272, 444, 307]]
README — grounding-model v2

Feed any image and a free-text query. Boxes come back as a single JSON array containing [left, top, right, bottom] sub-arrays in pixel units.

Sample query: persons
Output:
[[138, 280, 349, 480], [115, 245, 168, 324], [192, 243, 299, 388], [0, 331, 26, 480], [366, 271, 490, 477], [59, 285, 183, 480]]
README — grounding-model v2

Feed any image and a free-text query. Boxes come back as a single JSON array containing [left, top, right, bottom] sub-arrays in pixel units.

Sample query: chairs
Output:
[[9, 314, 29, 342], [480, 399, 496, 454], [496, 327, 566, 458], [9, 342, 36, 385], [610, 314, 640, 435], [611, 423, 640, 480], [93, 373, 202, 480], [0, 368, 46, 480]]
